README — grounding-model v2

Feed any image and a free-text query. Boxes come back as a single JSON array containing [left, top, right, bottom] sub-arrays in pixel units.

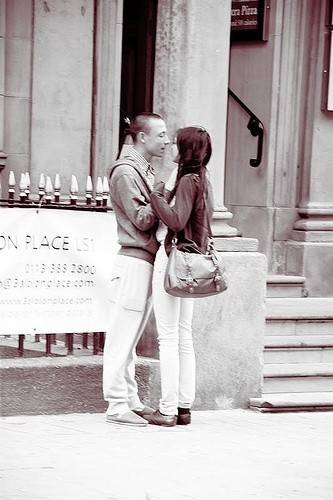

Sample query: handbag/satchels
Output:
[[164, 244, 227, 298]]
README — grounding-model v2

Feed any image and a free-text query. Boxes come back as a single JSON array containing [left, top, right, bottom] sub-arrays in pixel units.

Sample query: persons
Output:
[[143, 127, 214, 427], [103, 112, 178, 427]]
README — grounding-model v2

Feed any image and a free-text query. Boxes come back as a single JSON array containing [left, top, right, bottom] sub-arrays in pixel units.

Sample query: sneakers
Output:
[[178, 410, 191, 425], [105, 405, 155, 427], [143, 409, 177, 427]]
